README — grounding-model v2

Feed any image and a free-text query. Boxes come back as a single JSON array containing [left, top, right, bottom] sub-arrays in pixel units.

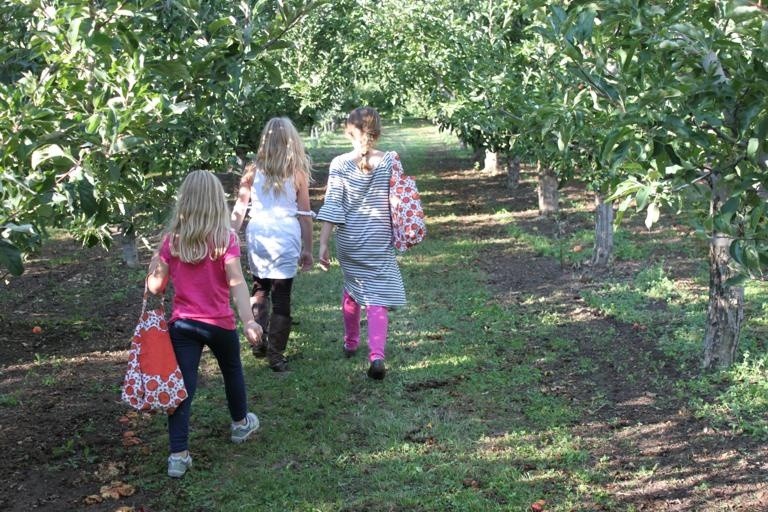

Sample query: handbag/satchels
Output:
[[387, 151, 427, 252], [118, 305, 189, 417]]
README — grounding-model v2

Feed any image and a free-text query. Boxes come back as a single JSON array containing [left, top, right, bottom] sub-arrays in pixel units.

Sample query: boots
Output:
[[265, 314, 295, 371], [250, 304, 268, 358]]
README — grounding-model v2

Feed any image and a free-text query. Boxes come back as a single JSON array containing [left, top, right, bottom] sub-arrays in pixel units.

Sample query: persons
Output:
[[310, 106, 408, 381], [145, 170, 262, 479], [229, 117, 313, 373]]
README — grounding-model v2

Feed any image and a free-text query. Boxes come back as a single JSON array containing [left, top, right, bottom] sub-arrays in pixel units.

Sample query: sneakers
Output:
[[167, 449, 192, 478], [367, 359, 386, 379], [230, 412, 261, 444]]
